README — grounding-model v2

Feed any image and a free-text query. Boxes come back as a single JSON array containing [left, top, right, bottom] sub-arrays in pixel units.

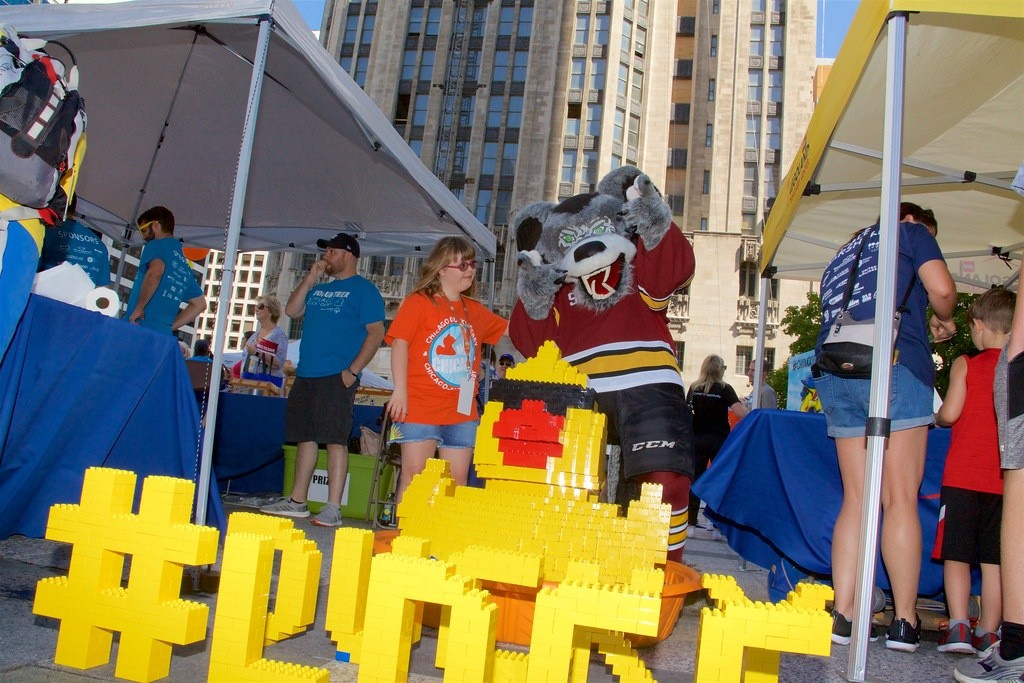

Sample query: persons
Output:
[[954, 160, 1024, 683], [931, 288, 1017, 658], [467, 343, 515, 489], [686, 354, 778, 542], [0, 35, 87, 366], [36, 191, 111, 289], [384, 235, 511, 530], [177, 295, 288, 398], [122, 206, 207, 336], [811, 202, 958, 652]]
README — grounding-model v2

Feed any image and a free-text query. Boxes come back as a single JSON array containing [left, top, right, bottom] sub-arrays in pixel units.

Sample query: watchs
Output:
[[254, 350, 260, 357]]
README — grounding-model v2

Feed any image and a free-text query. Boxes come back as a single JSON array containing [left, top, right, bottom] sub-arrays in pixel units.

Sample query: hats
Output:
[[317, 233, 361, 259], [499, 354, 515, 362]]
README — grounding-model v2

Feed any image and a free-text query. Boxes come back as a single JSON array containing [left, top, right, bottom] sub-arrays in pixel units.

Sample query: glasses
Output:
[[500, 361, 513, 366], [256, 303, 270, 310], [721, 365, 727, 370], [443, 260, 476, 272], [137, 220, 158, 236]]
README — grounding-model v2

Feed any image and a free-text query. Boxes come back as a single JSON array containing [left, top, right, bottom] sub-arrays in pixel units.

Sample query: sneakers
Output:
[[937, 623, 999, 657], [954, 645, 1024, 683], [832, 611, 879, 645], [259, 496, 310, 518], [886, 613, 922, 652], [312, 506, 343, 527]]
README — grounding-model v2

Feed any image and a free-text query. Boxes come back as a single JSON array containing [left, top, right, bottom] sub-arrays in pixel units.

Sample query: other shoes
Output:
[[711, 528, 721, 539], [707, 521, 713, 531], [687, 526, 696, 538]]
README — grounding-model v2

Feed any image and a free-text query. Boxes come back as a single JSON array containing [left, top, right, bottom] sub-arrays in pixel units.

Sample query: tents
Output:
[[222, 337, 395, 396], [751, 0, 1024, 683], [0, 0, 497, 591], [260, 232, 385, 527]]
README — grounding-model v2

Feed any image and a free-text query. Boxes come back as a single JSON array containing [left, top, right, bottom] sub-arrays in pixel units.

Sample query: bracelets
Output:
[[347, 367, 358, 378]]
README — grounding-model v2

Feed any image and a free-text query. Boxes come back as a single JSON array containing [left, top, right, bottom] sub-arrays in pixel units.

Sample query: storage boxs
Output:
[[280, 442, 391, 519], [232, 377, 280, 396]]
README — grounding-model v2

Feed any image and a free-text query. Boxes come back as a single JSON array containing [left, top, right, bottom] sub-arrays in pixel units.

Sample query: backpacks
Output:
[[0, 24, 88, 211]]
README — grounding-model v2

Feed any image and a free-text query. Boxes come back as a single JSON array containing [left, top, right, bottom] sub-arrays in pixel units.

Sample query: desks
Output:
[[216, 393, 388, 508], [690, 407, 980, 619], [0, 301, 219, 545]]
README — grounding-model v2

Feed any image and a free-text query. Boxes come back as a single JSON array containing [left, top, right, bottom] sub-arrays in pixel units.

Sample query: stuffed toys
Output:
[[509, 166, 695, 563]]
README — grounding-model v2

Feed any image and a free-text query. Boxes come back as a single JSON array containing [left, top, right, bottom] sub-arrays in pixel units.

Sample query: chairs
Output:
[[185, 360, 229, 390], [363, 396, 402, 529]]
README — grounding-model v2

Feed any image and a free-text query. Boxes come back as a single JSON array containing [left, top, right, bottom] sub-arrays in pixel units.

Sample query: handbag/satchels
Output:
[[816, 310, 903, 378], [241, 352, 283, 388]]
